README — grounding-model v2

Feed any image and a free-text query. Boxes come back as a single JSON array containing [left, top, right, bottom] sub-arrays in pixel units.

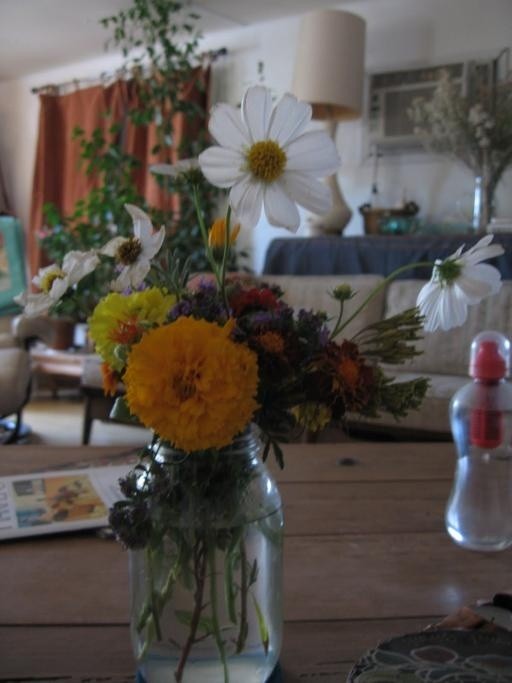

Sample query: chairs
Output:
[[261, 234, 512, 443]]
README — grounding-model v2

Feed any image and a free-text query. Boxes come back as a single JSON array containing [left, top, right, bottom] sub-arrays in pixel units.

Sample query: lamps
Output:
[[291, 10, 364, 235]]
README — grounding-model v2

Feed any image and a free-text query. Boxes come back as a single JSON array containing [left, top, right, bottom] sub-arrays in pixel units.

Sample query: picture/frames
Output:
[[366, 59, 475, 158]]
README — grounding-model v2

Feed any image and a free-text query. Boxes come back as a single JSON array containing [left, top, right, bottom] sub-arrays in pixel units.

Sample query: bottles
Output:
[[443, 330, 512, 552]]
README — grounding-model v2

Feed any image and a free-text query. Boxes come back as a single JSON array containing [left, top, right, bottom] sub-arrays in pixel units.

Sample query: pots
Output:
[[358, 200, 419, 235]]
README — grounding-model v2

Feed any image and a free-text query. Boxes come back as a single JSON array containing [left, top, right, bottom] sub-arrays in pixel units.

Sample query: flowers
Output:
[[15, 83, 503, 683], [407, 68, 512, 174]]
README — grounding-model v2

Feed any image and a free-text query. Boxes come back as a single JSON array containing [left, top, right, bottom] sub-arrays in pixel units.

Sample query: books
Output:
[[0, 448, 154, 543]]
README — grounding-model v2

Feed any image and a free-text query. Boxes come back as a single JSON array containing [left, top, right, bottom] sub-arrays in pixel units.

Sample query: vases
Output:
[[472, 174, 487, 233]]
